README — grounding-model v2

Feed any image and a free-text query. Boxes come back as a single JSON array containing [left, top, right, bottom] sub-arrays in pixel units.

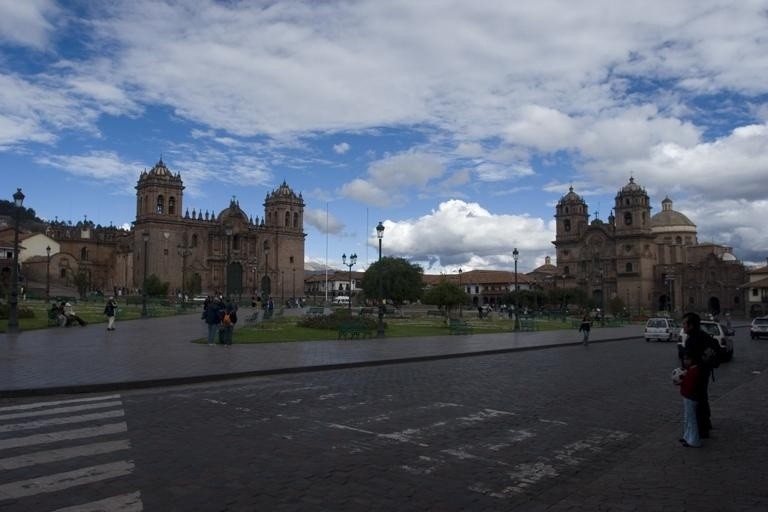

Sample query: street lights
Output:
[[44, 244, 53, 305], [139, 229, 150, 318], [342, 252, 358, 321], [562, 272, 568, 324], [458, 267, 465, 318], [175, 240, 195, 304], [252, 245, 297, 306], [512, 246, 522, 330], [374, 220, 387, 338], [6, 186, 27, 334]]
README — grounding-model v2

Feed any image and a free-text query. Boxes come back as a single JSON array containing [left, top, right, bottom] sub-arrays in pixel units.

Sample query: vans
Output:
[[332, 295, 353, 305]]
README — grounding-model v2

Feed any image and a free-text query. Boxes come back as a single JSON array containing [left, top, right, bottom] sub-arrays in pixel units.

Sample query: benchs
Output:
[[48, 309, 71, 326], [244, 307, 621, 339]]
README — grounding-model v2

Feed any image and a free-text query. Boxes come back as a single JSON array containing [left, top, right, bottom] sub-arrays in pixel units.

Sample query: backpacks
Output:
[[223, 311, 232, 327]]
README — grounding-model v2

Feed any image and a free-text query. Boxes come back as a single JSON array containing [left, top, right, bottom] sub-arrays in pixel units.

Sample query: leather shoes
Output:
[[678, 439, 690, 447]]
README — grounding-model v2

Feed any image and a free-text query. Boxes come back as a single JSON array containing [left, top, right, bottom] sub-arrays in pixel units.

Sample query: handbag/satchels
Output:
[[702, 337, 723, 371]]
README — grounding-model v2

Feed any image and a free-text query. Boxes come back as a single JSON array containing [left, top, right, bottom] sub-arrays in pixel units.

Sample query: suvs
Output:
[[677, 319, 734, 362], [749, 315, 768, 340]]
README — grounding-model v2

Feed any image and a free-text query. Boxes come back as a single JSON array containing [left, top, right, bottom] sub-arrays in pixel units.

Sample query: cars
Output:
[[643, 318, 682, 343]]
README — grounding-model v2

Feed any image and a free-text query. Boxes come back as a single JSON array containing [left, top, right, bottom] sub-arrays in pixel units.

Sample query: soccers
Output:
[[671, 367, 687, 385]]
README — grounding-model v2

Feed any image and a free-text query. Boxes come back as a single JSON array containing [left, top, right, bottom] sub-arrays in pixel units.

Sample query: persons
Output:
[[579, 315, 591, 345], [680, 351, 704, 447], [678, 310, 714, 438], [201, 295, 238, 347], [52, 302, 89, 328], [252, 296, 304, 315], [103, 296, 117, 330]]
